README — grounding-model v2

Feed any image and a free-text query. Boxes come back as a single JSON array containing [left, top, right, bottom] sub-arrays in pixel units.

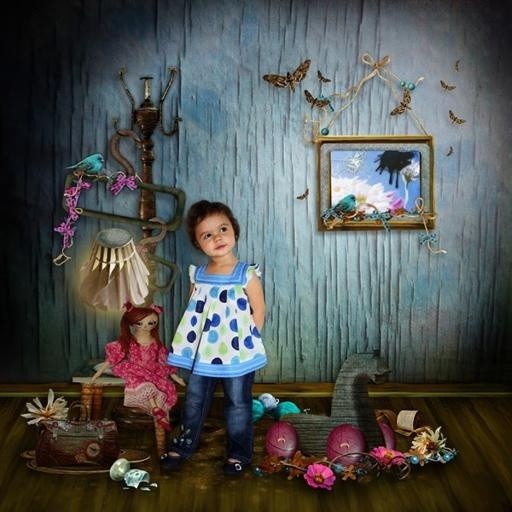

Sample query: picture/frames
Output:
[[314, 131, 437, 234]]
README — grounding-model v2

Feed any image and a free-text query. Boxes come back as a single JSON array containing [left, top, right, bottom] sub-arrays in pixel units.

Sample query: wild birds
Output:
[[334, 194, 357, 212], [64, 153, 105, 176]]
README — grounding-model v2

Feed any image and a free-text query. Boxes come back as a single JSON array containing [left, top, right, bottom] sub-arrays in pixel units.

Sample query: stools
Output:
[[68, 374, 173, 458]]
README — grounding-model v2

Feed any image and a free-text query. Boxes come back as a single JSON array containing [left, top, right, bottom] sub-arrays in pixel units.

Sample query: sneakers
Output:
[[159, 449, 188, 473], [153, 406, 172, 432], [222, 454, 254, 476]]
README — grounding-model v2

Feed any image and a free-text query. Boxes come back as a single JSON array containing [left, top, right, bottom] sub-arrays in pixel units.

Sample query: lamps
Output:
[[76, 214, 166, 313]]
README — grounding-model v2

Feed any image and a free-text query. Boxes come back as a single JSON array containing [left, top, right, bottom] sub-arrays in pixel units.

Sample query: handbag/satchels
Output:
[[34, 400, 122, 474]]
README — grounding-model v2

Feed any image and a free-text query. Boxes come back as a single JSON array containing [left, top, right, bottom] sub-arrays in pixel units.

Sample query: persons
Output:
[[87, 301, 187, 458], [160, 200, 269, 476]]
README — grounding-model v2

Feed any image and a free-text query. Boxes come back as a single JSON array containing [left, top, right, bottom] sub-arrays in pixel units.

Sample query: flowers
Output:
[[280, 424, 452, 491]]
